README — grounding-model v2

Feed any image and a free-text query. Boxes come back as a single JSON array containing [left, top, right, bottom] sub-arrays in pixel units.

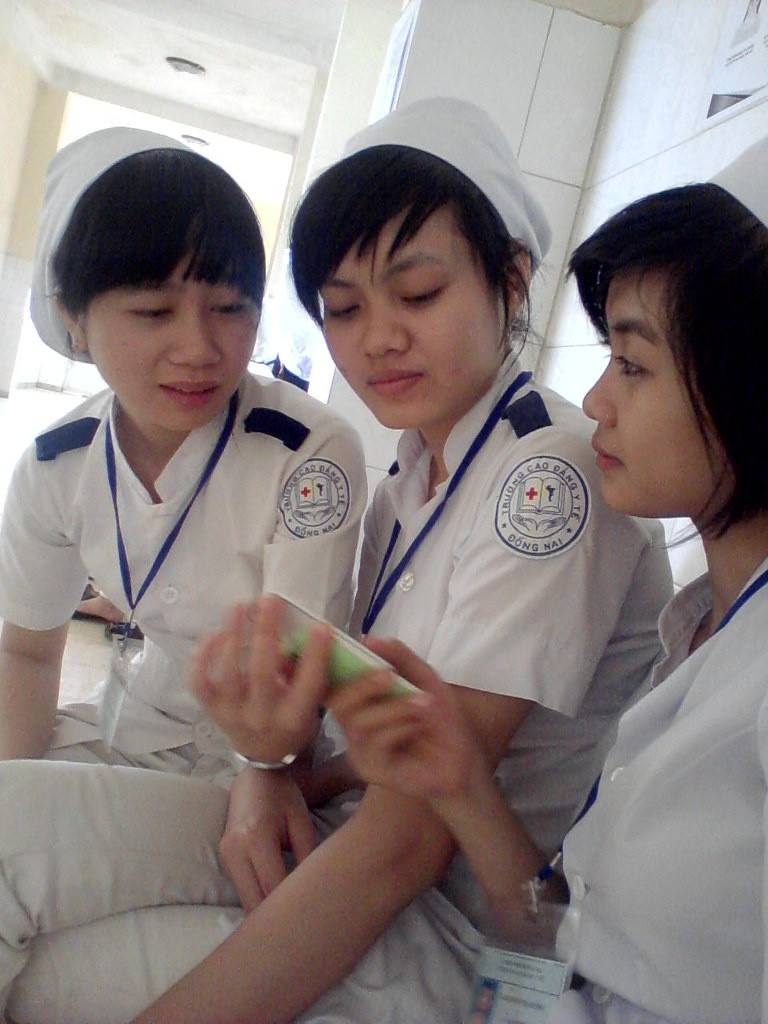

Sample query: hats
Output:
[[339, 101, 554, 279], [31, 120, 192, 363], [709, 137, 768, 235]]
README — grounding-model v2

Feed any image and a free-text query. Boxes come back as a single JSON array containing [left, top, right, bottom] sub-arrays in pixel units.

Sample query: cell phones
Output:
[[244, 592, 425, 703]]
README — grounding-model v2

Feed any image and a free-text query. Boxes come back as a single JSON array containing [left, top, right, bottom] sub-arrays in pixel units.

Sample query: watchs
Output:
[[232, 739, 318, 778]]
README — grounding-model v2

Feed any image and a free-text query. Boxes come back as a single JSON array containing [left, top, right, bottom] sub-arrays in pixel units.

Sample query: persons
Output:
[[5, 119, 371, 846], [317, 135, 768, 1024], [2, 88, 675, 1020], [76, 331, 323, 624]]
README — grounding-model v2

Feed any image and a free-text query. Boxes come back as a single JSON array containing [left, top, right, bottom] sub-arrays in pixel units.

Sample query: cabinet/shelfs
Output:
[[317, 0, 624, 581]]
